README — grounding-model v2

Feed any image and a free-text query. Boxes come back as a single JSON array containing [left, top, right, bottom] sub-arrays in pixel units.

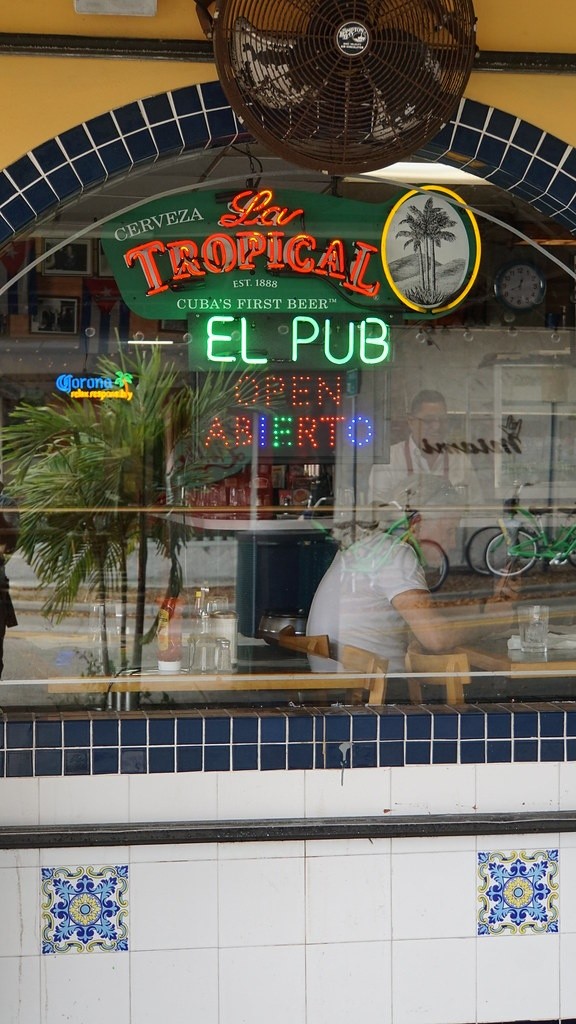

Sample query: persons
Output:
[[39, 306, 71, 331], [0, 483, 20, 678], [369, 390, 486, 507], [305, 476, 516, 698], [65, 246, 78, 269]]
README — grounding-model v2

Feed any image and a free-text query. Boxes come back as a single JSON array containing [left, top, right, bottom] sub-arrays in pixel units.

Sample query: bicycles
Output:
[[300, 495, 421, 595], [484, 508, 576, 577], [465, 482, 576, 577], [340, 485, 448, 593]]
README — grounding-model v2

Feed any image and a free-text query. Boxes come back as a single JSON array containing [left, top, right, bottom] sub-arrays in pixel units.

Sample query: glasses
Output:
[[410, 413, 449, 426], [407, 482, 458, 522]]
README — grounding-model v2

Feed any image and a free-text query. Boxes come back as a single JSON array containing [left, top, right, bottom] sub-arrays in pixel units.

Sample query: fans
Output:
[[193, 0, 476, 175]]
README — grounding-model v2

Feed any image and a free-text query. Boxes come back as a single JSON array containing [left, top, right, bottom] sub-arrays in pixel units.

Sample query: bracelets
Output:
[[480, 603, 484, 613]]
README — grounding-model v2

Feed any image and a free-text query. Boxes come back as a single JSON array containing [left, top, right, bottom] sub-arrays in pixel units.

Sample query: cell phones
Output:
[[499, 557, 520, 598]]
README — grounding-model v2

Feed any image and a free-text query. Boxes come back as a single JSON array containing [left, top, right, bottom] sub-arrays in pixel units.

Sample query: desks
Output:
[[461, 635, 576, 700]]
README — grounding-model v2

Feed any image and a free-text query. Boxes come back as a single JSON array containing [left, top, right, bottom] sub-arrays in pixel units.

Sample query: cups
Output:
[[196, 486, 248, 519], [516, 606, 549, 653]]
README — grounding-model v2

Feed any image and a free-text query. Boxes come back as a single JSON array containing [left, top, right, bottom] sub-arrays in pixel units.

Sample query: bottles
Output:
[[253, 465, 274, 519], [155, 588, 238, 674], [281, 498, 292, 515], [303, 464, 332, 507]]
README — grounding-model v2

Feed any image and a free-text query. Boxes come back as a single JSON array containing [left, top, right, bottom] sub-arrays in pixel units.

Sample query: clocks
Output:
[[494, 261, 545, 312]]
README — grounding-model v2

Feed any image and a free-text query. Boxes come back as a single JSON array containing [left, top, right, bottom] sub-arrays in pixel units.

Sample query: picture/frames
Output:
[[97, 238, 115, 280], [41, 237, 94, 278], [29, 296, 80, 335]]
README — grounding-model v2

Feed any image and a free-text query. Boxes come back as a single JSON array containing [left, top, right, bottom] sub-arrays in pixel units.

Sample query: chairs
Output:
[[276, 624, 473, 707]]
